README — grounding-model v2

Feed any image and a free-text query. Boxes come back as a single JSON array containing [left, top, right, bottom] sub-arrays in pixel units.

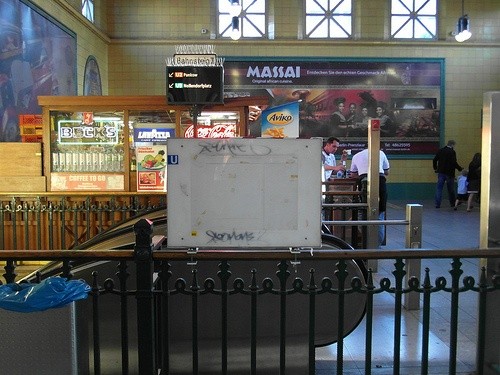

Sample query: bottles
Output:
[[342, 160, 346, 178]]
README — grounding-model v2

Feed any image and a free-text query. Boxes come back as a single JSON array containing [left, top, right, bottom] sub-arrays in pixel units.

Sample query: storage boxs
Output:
[[18, 114, 43, 136], [22, 135, 42, 143]]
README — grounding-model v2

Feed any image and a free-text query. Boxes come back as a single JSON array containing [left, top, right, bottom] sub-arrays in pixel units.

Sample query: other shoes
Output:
[[435, 205, 440, 208], [467, 208, 470, 211], [470, 205, 473, 209], [454, 206, 457, 210], [451, 204, 454, 207]]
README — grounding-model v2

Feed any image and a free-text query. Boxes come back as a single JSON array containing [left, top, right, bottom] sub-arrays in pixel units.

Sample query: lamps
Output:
[[231, 16, 241, 40], [231, 0, 241, 16], [454, 0, 472, 43]]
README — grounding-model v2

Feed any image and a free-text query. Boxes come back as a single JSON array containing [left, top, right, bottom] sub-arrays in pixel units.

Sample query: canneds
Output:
[[337, 169, 342, 178]]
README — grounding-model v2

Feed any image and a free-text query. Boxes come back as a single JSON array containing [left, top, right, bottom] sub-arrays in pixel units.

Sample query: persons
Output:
[[321, 137, 348, 233], [374, 106, 396, 138], [350, 143, 391, 248], [453, 168, 474, 211], [433, 140, 463, 208], [357, 103, 376, 137], [466, 152, 481, 211], [344, 103, 364, 137], [329, 97, 349, 137]]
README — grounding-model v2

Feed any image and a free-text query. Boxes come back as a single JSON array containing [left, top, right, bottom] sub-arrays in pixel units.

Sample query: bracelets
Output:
[[347, 124, 349, 128]]
